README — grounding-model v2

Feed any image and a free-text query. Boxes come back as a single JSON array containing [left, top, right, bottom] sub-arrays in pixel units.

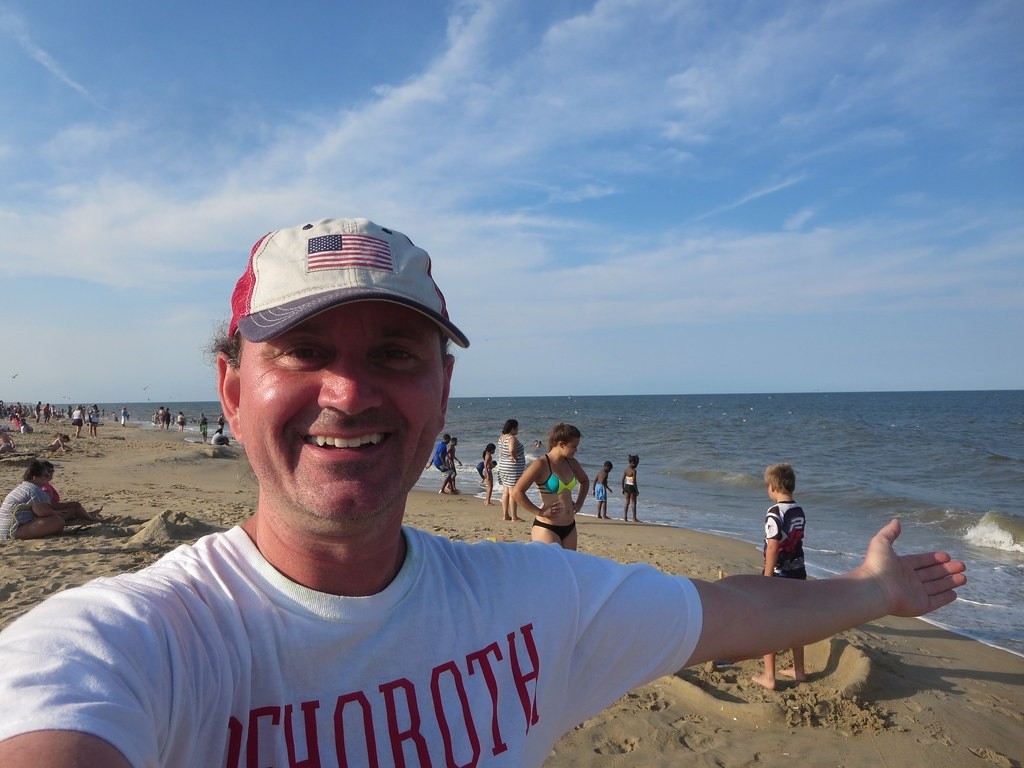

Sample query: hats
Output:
[[91, 404, 97, 407], [229, 217, 470, 348]]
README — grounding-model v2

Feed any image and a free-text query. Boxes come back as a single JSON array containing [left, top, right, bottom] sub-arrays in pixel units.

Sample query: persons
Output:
[[0, 400, 128, 439], [152, 407, 174, 429], [0, 217, 968, 768], [750, 462, 806, 690], [482, 443, 496, 505], [176, 411, 186, 432], [476, 460, 497, 482], [512, 423, 591, 550], [0, 430, 14, 448], [535, 439, 542, 447], [211, 430, 231, 446], [189, 412, 207, 435], [497, 419, 526, 522], [0, 459, 103, 540], [217, 413, 225, 431], [622, 454, 642, 522], [446, 437, 462, 489], [426, 434, 456, 494], [44, 435, 72, 451], [592, 461, 613, 519]]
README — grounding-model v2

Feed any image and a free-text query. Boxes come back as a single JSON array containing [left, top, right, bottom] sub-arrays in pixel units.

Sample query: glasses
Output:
[[41, 473, 50, 478]]
